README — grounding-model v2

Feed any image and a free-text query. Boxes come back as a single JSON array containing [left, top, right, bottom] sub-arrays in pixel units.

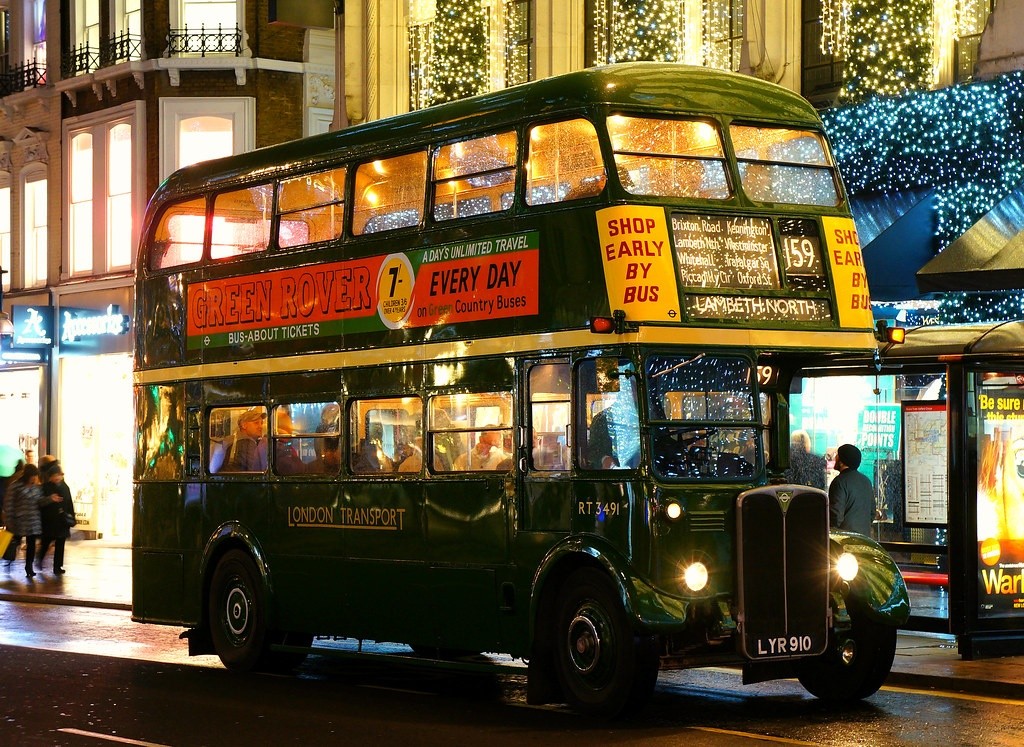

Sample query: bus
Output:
[[130, 58, 911, 719]]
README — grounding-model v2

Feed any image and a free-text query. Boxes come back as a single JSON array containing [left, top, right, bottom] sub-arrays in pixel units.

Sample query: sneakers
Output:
[[36, 563, 45, 570], [52, 567, 66, 574]]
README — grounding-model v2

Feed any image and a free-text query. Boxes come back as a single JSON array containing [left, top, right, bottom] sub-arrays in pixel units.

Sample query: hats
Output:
[[37, 455, 59, 469], [47, 465, 65, 477], [237, 411, 266, 426]]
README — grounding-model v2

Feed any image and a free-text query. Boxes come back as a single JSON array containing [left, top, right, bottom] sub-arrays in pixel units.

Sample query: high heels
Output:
[[25, 565, 37, 576]]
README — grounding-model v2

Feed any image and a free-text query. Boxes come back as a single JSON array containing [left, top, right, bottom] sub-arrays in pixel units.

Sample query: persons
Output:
[[563, 164, 634, 203], [664, 159, 711, 198], [5, 459, 24, 486], [741, 163, 779, 204], [788, 430, 823, 488], [590, 381, 675, 473], [826, 445, 876, 539], [2, 463, 43, 577], [203, 407, 570, 474], [35, 465, 77, 575], [25, 449, 35, 469]]
